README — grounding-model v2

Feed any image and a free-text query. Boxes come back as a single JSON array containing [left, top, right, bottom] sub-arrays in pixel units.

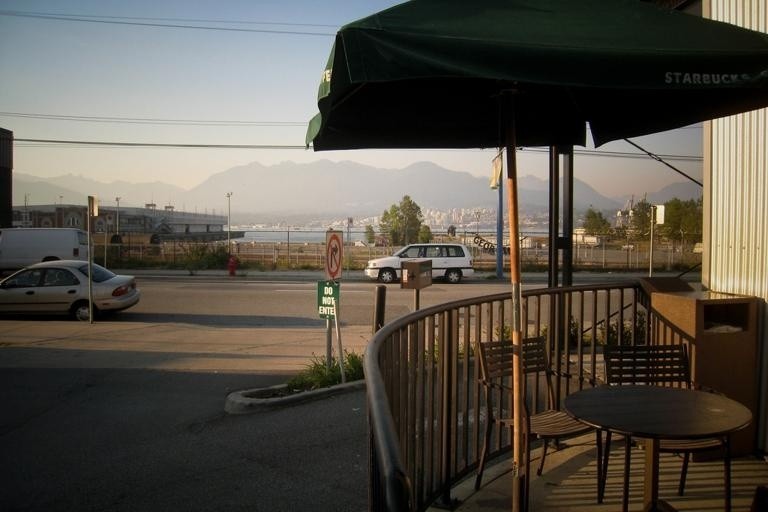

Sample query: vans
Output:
[[365, 244, 475, 284]]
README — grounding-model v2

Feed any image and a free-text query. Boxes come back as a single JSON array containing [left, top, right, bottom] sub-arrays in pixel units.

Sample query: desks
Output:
[[561, 383, 757, 510]]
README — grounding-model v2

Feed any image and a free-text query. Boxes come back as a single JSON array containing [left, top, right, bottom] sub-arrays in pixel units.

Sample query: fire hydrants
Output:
[[227, 257, 237, 277]]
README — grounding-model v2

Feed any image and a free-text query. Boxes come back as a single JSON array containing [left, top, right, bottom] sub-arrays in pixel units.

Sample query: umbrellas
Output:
[[306, 0, 768, 512]]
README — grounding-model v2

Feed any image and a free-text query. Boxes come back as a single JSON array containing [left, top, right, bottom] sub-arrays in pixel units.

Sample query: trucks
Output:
[[0, 226, 94, 276]]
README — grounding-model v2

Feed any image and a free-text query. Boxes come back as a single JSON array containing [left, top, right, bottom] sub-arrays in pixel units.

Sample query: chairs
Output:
[[473, 336, 596, 490], [600, 344, 729, 495]]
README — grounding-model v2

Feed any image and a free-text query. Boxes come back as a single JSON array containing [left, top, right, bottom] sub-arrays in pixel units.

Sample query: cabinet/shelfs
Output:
[[648, 288, 762, 465]]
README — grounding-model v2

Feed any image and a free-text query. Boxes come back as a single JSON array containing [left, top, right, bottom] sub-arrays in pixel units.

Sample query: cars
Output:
[[620, 243, 635, 251], [1, 259, 141, 324]]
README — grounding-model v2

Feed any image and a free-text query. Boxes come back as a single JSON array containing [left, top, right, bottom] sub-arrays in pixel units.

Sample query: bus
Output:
[[692, 243, 702, 254]]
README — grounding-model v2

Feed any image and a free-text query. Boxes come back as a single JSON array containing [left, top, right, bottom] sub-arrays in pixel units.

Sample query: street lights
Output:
[[223, 191, 233, 256], [116, 196, 122, 234], [474, 210, 481, 257]]
